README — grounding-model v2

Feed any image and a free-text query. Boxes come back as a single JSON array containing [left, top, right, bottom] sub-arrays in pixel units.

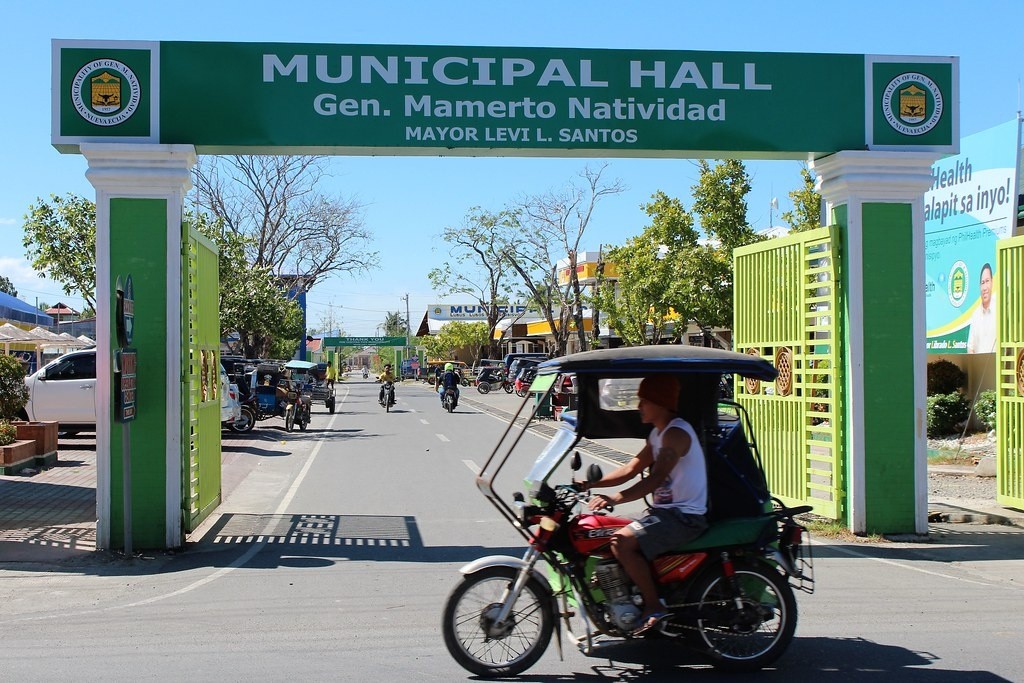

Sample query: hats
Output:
[[638, 374, 680, 411]]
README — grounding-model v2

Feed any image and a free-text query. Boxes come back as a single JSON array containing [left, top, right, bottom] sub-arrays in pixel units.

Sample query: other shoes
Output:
[[392, 402, 396, 404], [379, 400, 382, 404]]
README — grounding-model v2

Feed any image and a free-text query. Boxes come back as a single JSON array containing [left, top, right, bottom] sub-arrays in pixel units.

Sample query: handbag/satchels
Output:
[[710, 420, 770, 518]]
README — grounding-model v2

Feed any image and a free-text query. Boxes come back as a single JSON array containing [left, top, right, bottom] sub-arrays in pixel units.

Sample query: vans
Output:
[[427, 360, 472, 384]]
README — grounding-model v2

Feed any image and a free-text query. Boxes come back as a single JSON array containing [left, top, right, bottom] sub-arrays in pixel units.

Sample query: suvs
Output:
[[10, 344, 244, 424]]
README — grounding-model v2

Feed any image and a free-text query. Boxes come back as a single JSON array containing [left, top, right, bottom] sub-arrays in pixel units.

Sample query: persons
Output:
[[437, 363, 461, 408], [361, 365, 370, 378], [417, 366, 422, 376], [966, 263, 997, 355], [454, 369, 465, 385], [379, 364, 397, 405], [573, 375, 714, 637], [325, 361, 338, 389], [434, 364, 441, 393], [504, 366, 508, 375]]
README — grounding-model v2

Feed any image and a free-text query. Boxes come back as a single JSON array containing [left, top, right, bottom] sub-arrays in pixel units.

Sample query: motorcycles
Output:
[[475, 366, 514, 395], [361, 369, 370, 380], [230, 363, 258, 431], [439, 343, 815, 678], [377, 381, 397, 413], [279, 359, 320, 433], [439, 381, 461, 415], [451, 367, 470, 387]]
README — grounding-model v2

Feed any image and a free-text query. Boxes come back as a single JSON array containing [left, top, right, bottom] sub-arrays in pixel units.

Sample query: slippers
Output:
[[632, 612, 675, 635]]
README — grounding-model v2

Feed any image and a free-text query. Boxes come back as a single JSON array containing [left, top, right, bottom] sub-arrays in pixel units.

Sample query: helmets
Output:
[[445, 363, 453, 371]]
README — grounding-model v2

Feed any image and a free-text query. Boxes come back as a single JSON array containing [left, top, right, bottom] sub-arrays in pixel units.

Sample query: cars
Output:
[[220, 355, 337, 416], [477, 351, 583, 398]]
